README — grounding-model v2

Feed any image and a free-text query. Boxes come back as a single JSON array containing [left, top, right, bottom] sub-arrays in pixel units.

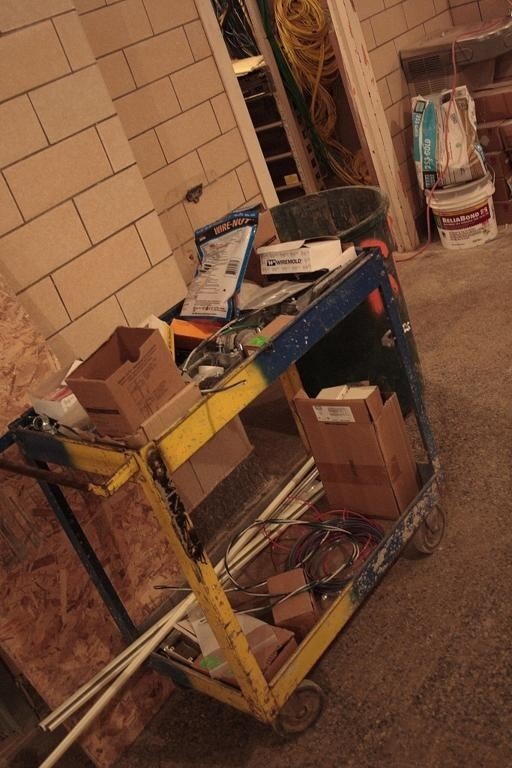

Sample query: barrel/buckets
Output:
[[422, 173, 498, 252]]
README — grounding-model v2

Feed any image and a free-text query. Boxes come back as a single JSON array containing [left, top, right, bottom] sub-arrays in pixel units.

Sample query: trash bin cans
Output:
[[270, 184, 428, 422]]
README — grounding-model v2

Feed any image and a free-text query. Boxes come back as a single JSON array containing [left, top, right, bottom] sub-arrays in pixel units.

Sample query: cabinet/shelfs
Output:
[[208, 2, 319, 201]]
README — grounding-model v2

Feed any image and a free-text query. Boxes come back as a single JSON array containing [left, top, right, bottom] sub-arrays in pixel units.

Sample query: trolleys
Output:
[[0, 243, 448, 739]]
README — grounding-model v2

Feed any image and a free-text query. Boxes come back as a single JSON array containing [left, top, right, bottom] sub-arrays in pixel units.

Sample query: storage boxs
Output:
[[292, 384, 422, 520], [65, 326, 205, 449], [266, 567, 318, 636], [176, 613, 297, 694], [256, 234, 341, 274]]
